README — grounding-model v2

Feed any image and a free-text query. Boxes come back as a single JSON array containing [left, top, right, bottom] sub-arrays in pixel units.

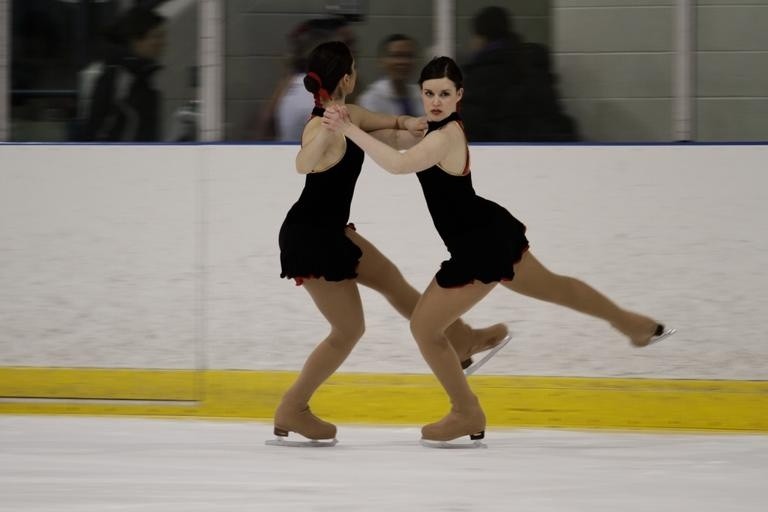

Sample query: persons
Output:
[[273, 41, 507, 441], [322, 57, 664, 440]]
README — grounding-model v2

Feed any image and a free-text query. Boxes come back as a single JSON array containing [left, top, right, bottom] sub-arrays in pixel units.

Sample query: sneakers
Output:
[[447, 324, 508, 369], [422, 402, 486, 441], [632, 314, 663, 346], [274, 404, 336, 439]]
